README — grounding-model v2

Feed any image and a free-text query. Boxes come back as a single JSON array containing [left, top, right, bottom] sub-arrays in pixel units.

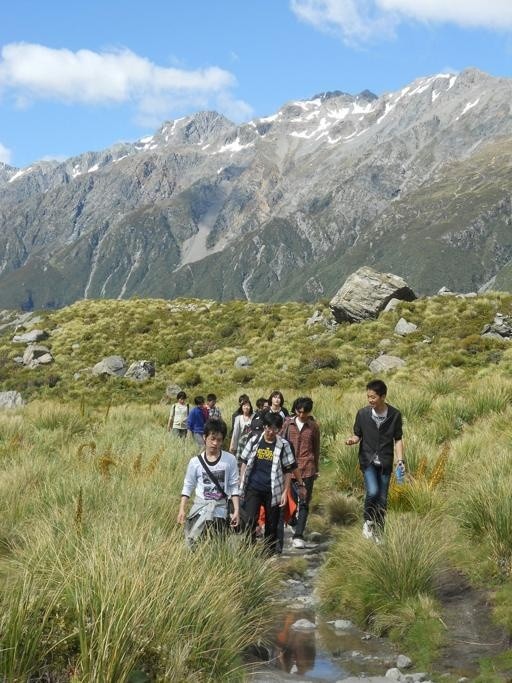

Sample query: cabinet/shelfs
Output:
[[396, 463, 403, 485]]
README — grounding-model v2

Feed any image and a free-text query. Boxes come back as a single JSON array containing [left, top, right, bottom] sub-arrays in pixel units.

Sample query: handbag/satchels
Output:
[[397, 459, 405, 464]]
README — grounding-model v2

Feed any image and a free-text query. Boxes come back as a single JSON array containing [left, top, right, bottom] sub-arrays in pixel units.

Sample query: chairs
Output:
[[286, 524, 297, 535], [362, 519, 384, 545], [293, 538, 305, 549]]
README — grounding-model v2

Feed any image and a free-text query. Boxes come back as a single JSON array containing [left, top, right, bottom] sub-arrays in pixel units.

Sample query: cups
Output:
[[265, 425, 279, 433]]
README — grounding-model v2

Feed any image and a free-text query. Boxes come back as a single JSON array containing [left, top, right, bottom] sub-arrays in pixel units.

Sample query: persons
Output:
[[167, 390, 320, 562], [344, 379, 405, 546]]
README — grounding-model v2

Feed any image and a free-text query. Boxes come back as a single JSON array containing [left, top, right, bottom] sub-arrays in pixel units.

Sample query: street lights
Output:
[[226, 499, 250, 535]]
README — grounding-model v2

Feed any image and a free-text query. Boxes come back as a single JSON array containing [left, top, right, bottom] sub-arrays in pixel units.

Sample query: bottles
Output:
[[251, 419, 263, 435]]
